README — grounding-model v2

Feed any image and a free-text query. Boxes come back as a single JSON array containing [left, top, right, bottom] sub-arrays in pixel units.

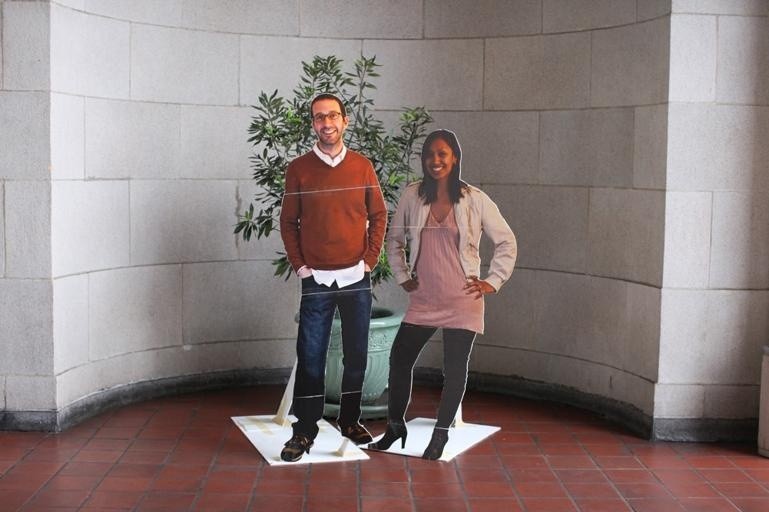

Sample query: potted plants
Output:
[[232, 52, 437, 421]]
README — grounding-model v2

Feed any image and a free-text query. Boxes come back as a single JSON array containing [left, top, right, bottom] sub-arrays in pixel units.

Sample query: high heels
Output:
[[368, 418, 407, 450], [422, 426, 448, 460]]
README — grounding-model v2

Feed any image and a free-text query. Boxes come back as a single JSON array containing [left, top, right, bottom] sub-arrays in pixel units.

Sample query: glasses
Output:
[[313, 110, 342, 121]]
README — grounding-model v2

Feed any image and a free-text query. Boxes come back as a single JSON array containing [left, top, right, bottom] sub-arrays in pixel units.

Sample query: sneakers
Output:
[[281, 434, 313, 461], [341, 422, 372, 444]]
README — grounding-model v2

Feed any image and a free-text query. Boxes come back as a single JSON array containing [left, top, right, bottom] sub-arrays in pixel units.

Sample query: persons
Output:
[[278, 94, 389, 462], [368, 128, 517, 460]]
